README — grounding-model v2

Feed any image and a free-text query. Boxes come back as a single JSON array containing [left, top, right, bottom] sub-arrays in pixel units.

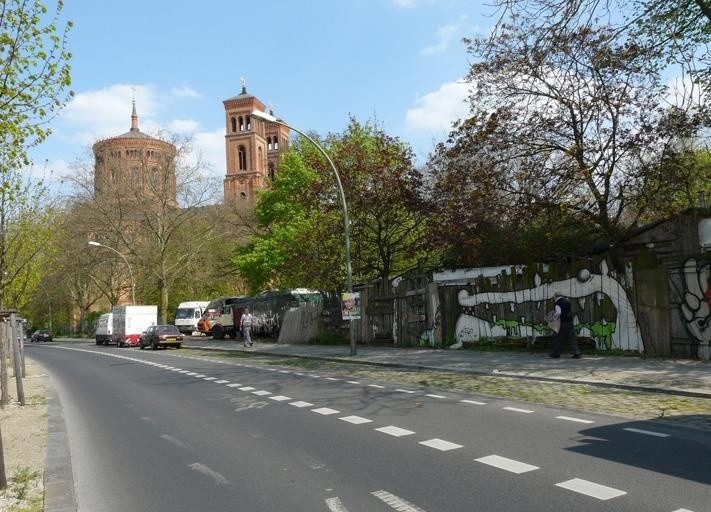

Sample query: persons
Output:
[[341, 293, 360, 318], [546, 290, 580, 359], [238, 308, 255, 348]]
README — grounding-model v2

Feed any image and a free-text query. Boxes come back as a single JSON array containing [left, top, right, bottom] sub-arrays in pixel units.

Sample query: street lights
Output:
[[89, 240, 136, 305], [249, 110, 358, 356]]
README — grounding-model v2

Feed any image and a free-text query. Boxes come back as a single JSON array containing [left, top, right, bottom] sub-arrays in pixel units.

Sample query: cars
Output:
[[30, 330, 52, 342], [138, 324, 184, 349]]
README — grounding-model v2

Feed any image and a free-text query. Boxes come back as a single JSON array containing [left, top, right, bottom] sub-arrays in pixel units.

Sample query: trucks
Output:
[[175, 296, 245, 338], [95, 304, 159, 350]]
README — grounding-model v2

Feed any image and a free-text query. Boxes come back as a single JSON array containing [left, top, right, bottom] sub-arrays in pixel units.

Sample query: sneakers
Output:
[[547, 353, 583, 360], [249, 342, 253, 347]]
